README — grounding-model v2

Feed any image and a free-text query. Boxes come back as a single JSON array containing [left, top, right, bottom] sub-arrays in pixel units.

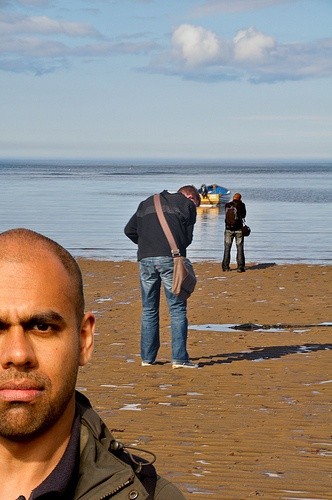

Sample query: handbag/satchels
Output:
[[169, 256, 196, 299], [242, 219, 250, 236]]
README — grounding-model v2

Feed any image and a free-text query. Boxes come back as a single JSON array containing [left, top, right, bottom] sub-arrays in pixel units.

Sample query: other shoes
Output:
[[172, 361, 199, 368], [223, 267, 230, 271], [141, 362, 154, 366], [237, 268, 245, 272]]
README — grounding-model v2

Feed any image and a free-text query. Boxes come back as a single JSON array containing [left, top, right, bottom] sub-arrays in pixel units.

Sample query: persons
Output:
[[222, 193, 246, 273], [199, 184, 207, 197], [0, 229, 158, 500], [124, 185, 201, 369], [211, 184, 217, 193]]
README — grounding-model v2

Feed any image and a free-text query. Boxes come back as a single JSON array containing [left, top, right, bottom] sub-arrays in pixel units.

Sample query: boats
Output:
[[197, 183, 231, 208]]
